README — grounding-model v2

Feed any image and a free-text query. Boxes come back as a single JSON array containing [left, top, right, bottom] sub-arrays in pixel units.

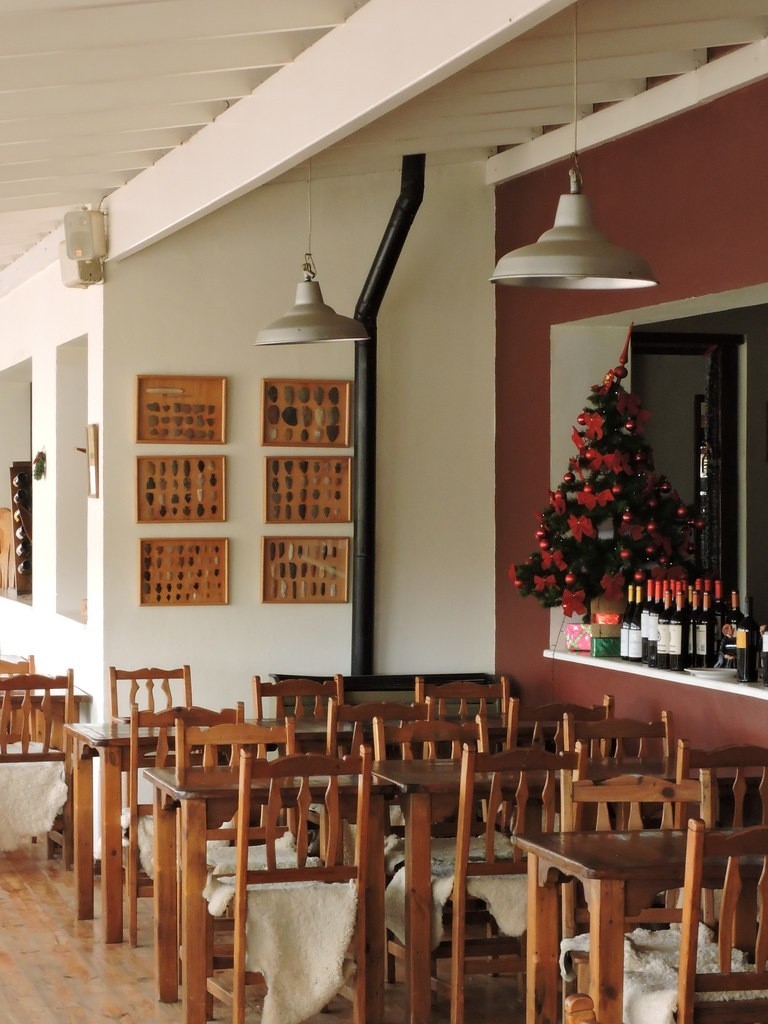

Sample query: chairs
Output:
[[0, 653, 768, 1024]]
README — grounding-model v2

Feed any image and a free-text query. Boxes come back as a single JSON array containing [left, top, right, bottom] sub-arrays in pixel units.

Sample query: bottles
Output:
[[621, 579, 757, 682]]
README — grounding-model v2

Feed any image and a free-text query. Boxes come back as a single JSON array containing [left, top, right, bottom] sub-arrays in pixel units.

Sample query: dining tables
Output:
[[372, 716, 586, 761], [142, 766, 398, 1024], [510, 826, 767, 1024], [370, 759, 609, 1023], [0, 675, 93, 871], [64, 715, 368, 943], [591, 757, 765, 830]]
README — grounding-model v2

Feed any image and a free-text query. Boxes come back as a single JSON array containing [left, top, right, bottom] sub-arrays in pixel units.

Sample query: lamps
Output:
[[254, 155, 372, 346], [490, 3, 658, 290]]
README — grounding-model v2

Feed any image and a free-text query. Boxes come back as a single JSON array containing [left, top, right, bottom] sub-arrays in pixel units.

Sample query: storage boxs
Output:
[[563, 592, 630, 657]]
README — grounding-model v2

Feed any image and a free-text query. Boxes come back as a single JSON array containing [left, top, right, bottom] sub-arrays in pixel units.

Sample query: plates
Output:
[[685, 668, 738, 680]]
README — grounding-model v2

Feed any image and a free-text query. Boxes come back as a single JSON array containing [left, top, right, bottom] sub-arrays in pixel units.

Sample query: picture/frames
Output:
[[264, 454, 354, 524], [135, 455, 228, 524], [261, 535, 350, 604], [134, 373, 228, 445], [138, 537, 230, 606], [260, 377, 352, 449], [84, 424, 99, 499]]
